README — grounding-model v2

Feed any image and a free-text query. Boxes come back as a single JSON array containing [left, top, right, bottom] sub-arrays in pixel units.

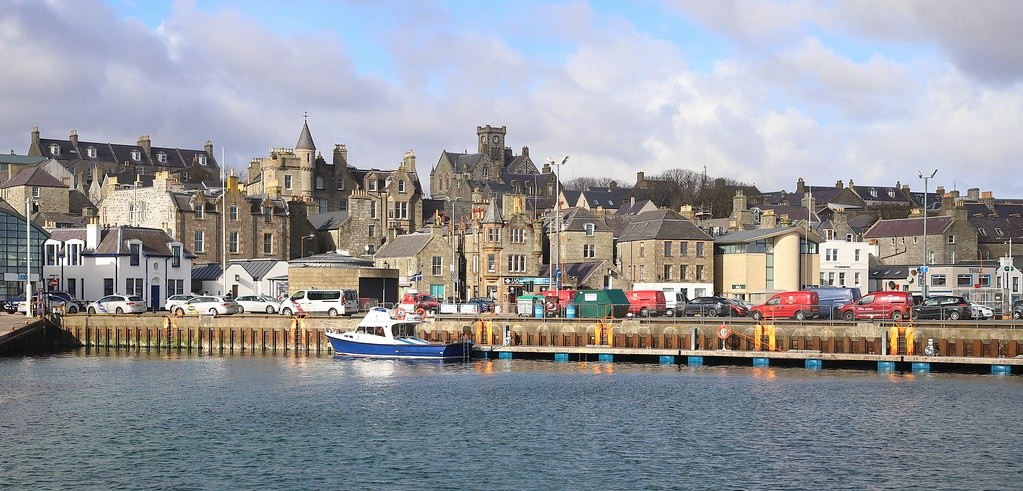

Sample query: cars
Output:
[[726, 298, 755, 317], [171, 296, 281, 316], [968, 300, 1023, 320], [18, 294, 146, 315]]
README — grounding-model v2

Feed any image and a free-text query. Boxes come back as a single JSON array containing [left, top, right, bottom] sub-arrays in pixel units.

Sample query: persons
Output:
[[226, 289, 232, 296]]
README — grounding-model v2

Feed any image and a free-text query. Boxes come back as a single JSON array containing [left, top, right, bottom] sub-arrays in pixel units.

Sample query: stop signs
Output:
[[51, 279, 58, 287]]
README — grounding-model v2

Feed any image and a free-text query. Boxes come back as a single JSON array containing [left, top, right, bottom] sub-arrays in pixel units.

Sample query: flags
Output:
[[412, 272, 421, 281]]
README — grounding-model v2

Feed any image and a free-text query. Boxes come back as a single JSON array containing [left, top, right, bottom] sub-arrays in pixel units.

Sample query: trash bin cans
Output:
[[516, 295, 538, 317]]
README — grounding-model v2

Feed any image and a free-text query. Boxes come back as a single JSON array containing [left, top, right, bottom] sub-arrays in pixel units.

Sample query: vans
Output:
[[663, 291, 685, 318], [624, 290, 666, 318], [750, 288, 971, 322], [279, 288, 359, 317], [534, 290, 577, 311]]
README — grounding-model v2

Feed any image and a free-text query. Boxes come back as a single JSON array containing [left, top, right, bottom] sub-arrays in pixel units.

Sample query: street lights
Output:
[[1004, 237, 1012, 312], [301, 234, 315, 258], [25, 197, 39, 317], [445, 196, 462, 303], [916, 169, 938, 301], [547, 155, 570, 298]]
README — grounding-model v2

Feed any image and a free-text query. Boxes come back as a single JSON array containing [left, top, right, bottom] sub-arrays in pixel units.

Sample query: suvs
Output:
[[165, 294, 195, 313], [4, 290, 72, 314], [677, 297, 730, 318], [403, 293, 497, 313]]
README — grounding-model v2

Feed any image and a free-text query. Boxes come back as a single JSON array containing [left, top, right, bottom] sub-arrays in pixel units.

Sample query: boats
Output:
[[324, 277, 474, 359]]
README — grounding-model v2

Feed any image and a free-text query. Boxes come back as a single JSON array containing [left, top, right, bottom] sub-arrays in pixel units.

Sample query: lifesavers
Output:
[[395, 307, 406, 320], [416, 308, 426, 320], [547, 301, 556, 310], [717, 325, 731, 339]]
